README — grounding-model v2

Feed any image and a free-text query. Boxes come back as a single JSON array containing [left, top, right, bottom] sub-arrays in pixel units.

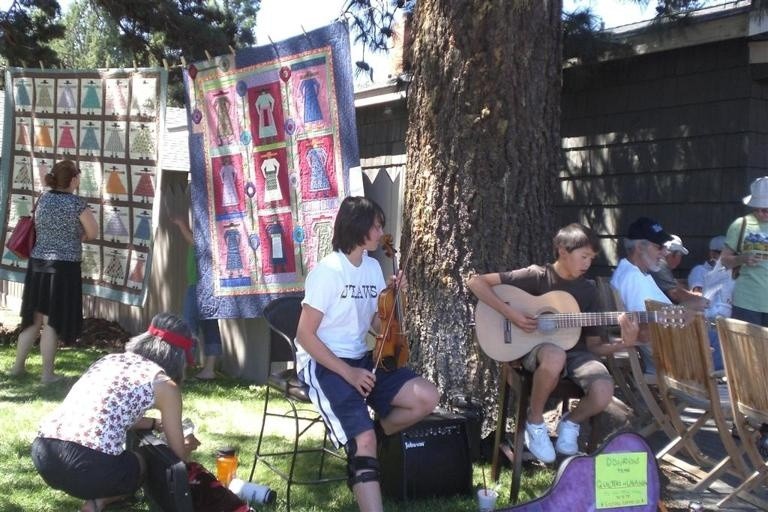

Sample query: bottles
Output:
[[215, 447, 235, 485], [228, 478, 278, 505]]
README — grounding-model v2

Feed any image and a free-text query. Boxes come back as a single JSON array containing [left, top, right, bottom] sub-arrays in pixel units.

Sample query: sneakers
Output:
[[523, 420, 558, 464], [555, 409, 582, 456]]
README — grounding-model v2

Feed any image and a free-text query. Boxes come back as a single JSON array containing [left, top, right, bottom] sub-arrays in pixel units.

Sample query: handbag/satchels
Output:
[[731, 251, 741, 280], [184, 460, 260, 512], [138, 443, 193, 511], [5, 214, 40, 257]]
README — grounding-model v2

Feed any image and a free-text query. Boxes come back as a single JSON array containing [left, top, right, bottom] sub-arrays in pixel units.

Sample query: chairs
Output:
[[593, 276, 694, 459], [643, 298, 759, 497], [491, 355, 602, 506], [248, 297, 349, 512], [713, 313, 768, 511]]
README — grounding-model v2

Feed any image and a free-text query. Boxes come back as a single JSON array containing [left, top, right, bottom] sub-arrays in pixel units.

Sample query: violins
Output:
[[373, 233, 409, 371]]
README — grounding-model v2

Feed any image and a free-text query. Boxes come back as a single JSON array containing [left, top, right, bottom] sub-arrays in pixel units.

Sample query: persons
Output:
[[467, 223, 639, 463], [610, 176, 768, 375], [8, 160, 98, 383], [294, 196, 439, 512], [31, 312, 201, 512]]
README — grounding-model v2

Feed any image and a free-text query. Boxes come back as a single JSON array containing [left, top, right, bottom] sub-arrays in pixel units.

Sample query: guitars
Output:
[[475, 284, 694, 363]]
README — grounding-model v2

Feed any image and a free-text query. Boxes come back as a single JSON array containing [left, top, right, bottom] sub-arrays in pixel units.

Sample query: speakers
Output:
[[381, 411, 472, 502]]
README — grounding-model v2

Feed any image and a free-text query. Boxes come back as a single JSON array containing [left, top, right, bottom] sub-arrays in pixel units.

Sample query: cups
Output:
[[476, 489, 500, 511]]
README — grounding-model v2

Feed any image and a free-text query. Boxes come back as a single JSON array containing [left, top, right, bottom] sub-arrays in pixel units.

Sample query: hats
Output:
[[625, 216, 674, 246], [662, 233, 690, 257], [146, 321, 199, 369], [741, 176, 768, 210], [708, 235, 727, 251]]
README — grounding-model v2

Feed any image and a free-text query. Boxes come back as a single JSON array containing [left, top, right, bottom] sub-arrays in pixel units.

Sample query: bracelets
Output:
[[151, 418, 156, 430]]
